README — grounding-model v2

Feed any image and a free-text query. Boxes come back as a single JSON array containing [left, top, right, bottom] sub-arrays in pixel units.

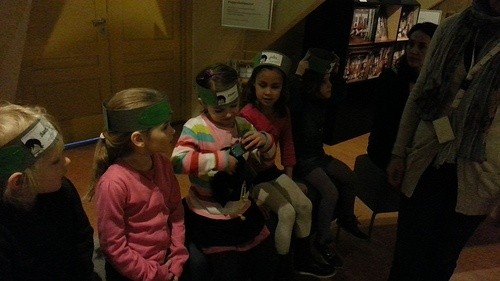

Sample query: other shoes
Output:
[[336, 214, 369, 241], [293, 253, 336, 278], [312, 244, 345, 270]]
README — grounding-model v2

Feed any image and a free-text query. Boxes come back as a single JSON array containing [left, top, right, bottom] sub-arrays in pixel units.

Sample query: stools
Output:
[[92, 154, 401, 281]]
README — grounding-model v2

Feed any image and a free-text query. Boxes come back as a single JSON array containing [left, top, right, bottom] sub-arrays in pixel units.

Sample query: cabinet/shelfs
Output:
[[265, 0, 421, 146]]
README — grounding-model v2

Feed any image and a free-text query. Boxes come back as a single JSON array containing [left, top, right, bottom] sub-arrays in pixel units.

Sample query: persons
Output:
[[237, 51, 338, 281], [171, 63, 291, 281], [0, 99, 103, 281], [286, 46, 369, 268], [80, 87, 202, 281], [387, 0, 500, 281], [366, 21, 437, 170]]
